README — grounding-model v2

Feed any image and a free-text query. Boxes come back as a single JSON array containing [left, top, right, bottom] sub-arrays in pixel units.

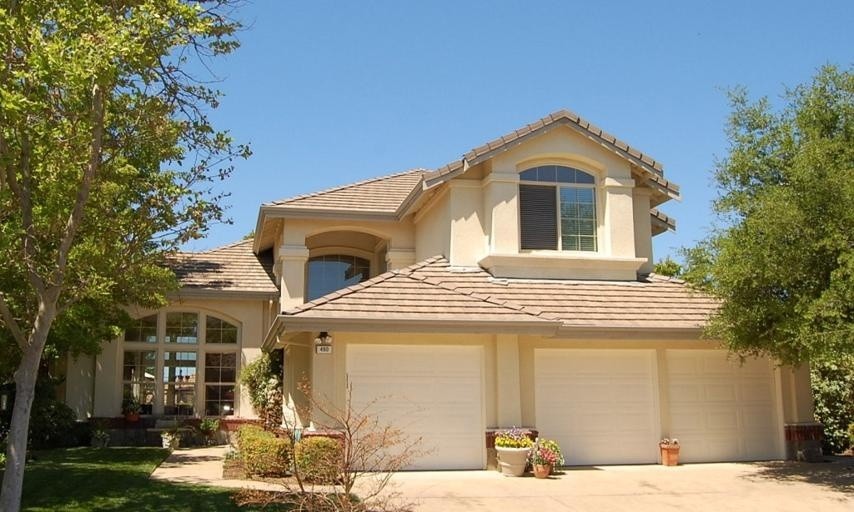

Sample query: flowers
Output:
[[494, 425, 531, 448], [526, 439, 564, 467], [659, 438, 679, 445]]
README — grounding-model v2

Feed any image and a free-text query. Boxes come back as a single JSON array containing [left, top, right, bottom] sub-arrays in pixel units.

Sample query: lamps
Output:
[[313, 331, 332, 346]]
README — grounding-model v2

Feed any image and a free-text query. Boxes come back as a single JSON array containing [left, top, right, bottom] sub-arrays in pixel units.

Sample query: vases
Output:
[[661, 444, 679, 466], [496, 446, 530, 477], [532, 463, 550, 478]]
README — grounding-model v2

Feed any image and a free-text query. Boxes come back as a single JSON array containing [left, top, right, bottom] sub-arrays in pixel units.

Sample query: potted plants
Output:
[[90, 428, 111, 447], [161, 431, 181, 448], [122, 401, 142, 422], [203, 418, 221, 439]]
